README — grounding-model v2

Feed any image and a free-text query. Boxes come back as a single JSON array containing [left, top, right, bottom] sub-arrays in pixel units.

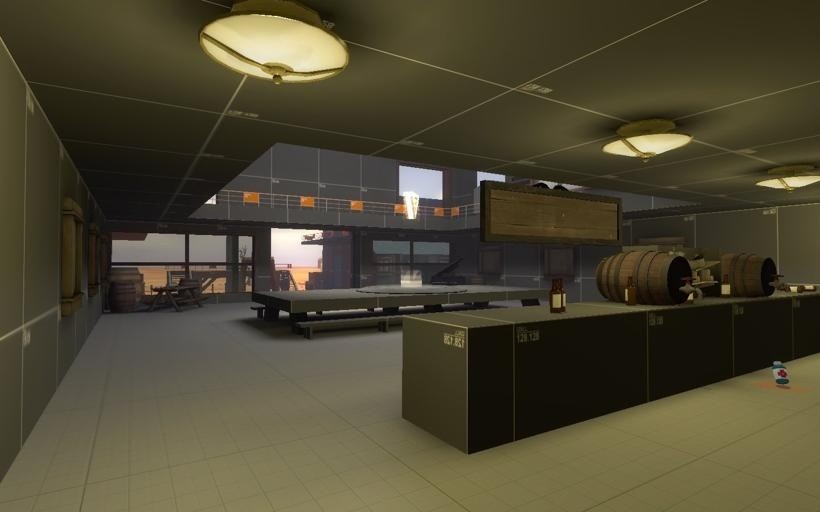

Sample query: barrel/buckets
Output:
[[719, 251, 778, 299], [106, 282, 140, 311], [594, 247, 693, 305]]
